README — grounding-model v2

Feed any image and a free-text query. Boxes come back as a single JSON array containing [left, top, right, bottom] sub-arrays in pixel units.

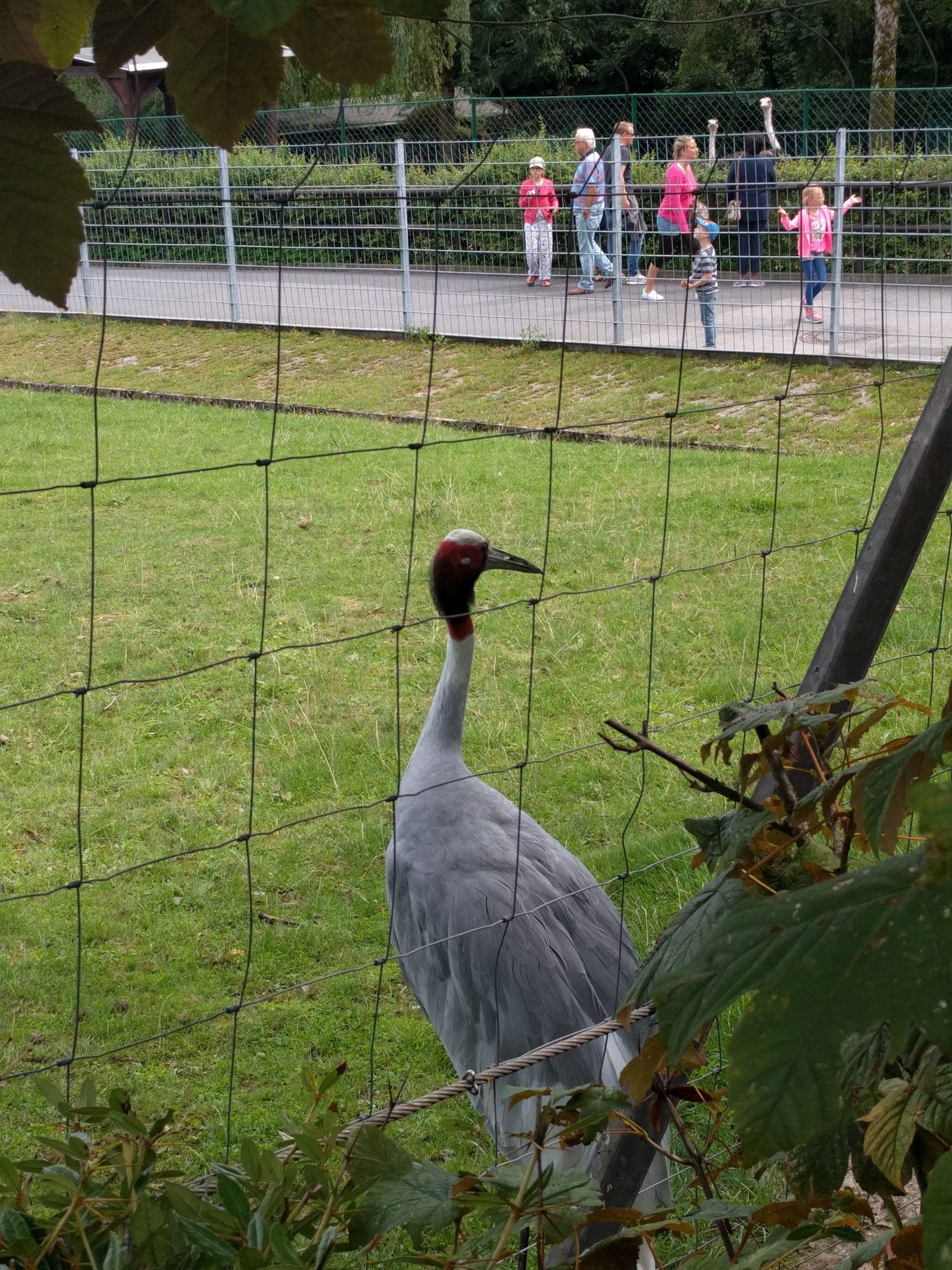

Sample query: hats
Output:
[[697, 217, 719, 241], [529, 157, 545, 168]]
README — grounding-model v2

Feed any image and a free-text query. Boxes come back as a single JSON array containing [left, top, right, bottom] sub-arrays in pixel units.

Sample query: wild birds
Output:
[[386, 528, 671, 1270]]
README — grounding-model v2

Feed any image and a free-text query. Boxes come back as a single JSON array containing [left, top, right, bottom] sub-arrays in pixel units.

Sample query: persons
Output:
[[518, 157, 558, 287], [602, 121, 647, 285], [567, 128, 614, 295], [681, 216, 720, 350], [724, 131, 778, 287], [641, 135, 702, 301], [778, 184, 862, 324]]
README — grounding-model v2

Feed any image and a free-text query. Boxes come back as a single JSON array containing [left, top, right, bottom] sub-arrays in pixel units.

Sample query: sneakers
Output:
[[642, 288, 664, 300], [527, 276, 535, 284], [541, 280, 550, 286], [622, 273, 627, 283], [748, 277, 765, 286], [627, 271, 648, 284], [805, 310, 824, 323], [733, 279, 747, 286], [802, 299, 809, 311], [694, 292, 699, 300]]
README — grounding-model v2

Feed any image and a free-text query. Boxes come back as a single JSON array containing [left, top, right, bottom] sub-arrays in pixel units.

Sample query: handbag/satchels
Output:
[[695, 201, 709, 223], [726, 199, 742, 222]]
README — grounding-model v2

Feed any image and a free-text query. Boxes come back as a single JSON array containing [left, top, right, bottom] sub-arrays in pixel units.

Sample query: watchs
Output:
[[582, 210, 589, 215]]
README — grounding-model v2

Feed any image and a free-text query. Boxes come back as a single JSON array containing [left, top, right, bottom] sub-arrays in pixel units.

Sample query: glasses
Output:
[[574, 141, 585, 146], [621, 132, 635, 137], [530, 167, 542, 172]]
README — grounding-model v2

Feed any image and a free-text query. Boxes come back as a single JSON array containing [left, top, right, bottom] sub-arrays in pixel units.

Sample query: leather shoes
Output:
[[568, 288, 594, 294], [605, 276, 613, 288]]
